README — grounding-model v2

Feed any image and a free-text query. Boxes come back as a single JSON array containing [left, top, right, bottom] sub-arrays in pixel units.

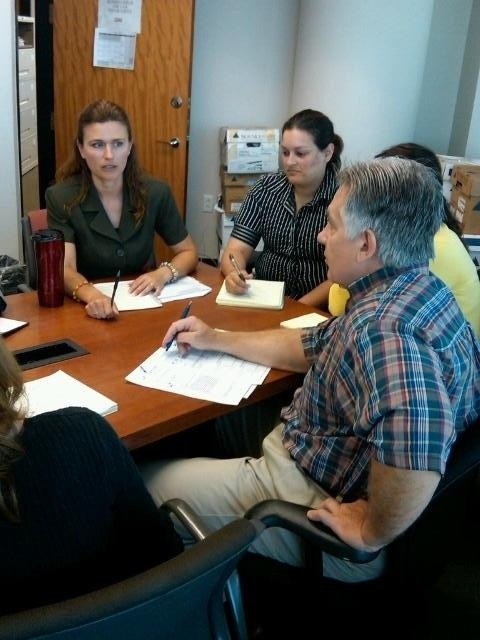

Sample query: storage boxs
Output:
[[223, 172, 263, 214], [218, 126, 279, 173], [437, 154, 470, 202], [446, 165, 479, 236], [219, 214, 264, 252]]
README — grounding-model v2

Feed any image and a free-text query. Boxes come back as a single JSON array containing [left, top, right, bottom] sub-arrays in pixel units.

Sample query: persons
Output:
[[45, 98, 199, 321], [220, 108, 346, 309], [328, 142, 480, 356], [138, 156, 480, 607], [0, 337, 184, 619]]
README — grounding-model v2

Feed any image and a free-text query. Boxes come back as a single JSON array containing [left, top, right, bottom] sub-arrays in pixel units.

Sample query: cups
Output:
[[31, 229, 65, 308]]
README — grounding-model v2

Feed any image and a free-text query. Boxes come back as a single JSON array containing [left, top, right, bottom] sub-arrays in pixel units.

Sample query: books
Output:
[[215, 278, 285, 310]]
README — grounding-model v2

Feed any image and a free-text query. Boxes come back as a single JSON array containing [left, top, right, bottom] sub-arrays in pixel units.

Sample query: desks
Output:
[[1, 261, 335, 458]]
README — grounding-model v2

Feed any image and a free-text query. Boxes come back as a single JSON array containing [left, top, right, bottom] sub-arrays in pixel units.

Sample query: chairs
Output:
[[1, 497, 253, 640], [18, 209, 46, 294], [246, 432, 479, 640]]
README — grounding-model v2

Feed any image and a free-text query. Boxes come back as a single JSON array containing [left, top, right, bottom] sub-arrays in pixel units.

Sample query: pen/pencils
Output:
[[165, 300, 193, 351], [229, 253, 249, 291], [111, 269, 120, 307]]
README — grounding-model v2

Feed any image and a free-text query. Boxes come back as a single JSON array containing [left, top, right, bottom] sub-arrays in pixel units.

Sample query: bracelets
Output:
[[160, 261, 179, 285], [72, 280, 92, 303]]
